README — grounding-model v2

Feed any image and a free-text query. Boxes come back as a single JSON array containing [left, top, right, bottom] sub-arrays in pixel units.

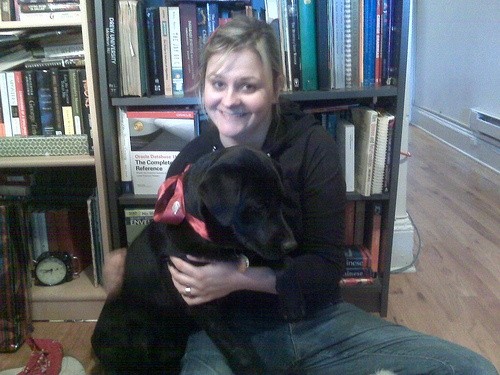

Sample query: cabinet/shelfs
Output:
[[0, 1, 412, 321]]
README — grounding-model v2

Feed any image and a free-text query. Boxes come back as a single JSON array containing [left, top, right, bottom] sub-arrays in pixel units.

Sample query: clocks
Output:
[[31, 251, 73, 287]]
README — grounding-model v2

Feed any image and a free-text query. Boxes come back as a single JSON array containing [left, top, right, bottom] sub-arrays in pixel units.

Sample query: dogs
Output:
[[90, 146, 297, 375]]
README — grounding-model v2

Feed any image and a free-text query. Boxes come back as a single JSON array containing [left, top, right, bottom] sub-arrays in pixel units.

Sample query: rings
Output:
[[185, 288, 192, 298]]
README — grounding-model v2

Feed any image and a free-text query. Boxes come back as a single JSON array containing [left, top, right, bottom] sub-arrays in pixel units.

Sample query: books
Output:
[[115, 108, 202, 246], [303, 103, 396, 196], [1, 170, 107, 353], [1, 0, 81, 21], [103, 1, 401, 98], [0, 27, 94, 158], [338, 200, 383, 287]]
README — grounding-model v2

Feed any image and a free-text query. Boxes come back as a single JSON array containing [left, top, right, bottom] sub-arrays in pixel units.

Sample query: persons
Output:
[[164, 15, 500, 375]]
